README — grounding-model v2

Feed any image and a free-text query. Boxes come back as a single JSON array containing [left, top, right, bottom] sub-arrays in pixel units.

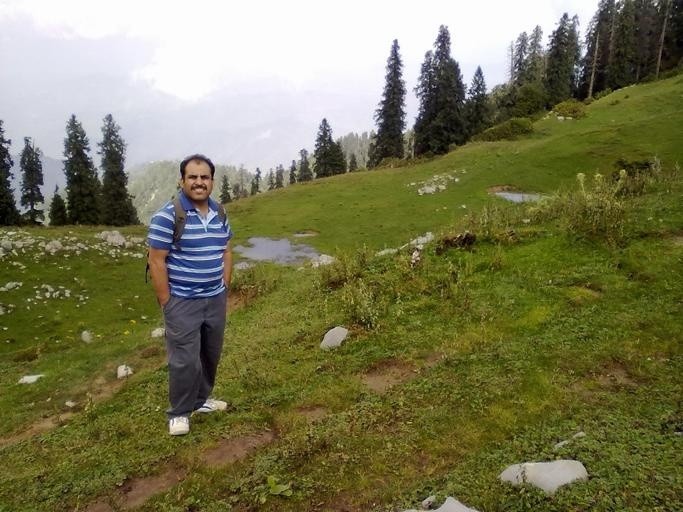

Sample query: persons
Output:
[[145, 153, 235, 437]]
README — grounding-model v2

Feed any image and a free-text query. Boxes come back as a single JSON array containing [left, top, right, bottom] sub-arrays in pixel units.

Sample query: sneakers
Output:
[[196, 399, 227, 412], [169, 416, 190, 436]]
